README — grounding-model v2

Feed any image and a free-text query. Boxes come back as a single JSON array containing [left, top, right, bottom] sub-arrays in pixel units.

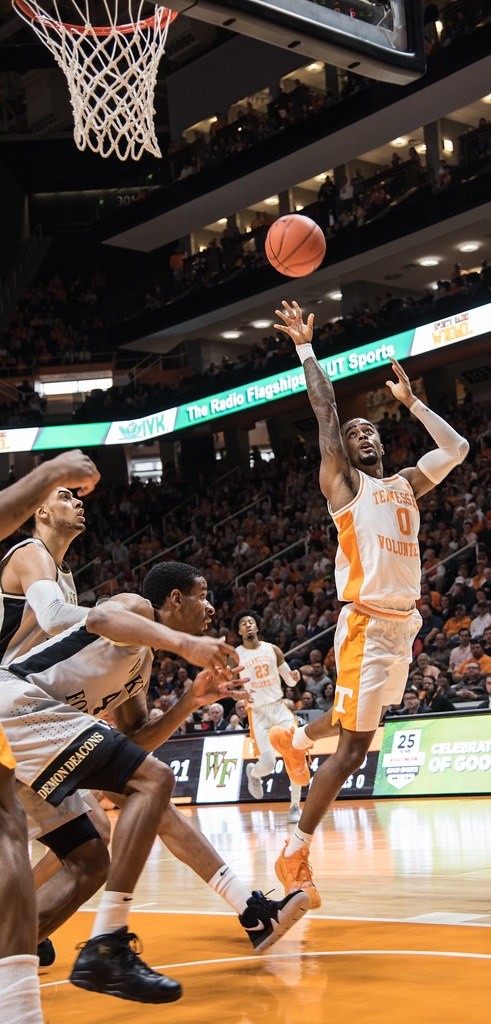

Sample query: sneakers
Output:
[[238, 889, 310, 953], [68, 925, 182, 1004], [287, 803, 301, 822], [36, 937, 56, 967], [274, 837, 321, 910], [268, 725, 314, 787], [245, 762, 264, 800]]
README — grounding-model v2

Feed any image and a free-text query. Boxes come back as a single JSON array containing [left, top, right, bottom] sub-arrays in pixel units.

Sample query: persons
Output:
[[1, 449, 306, 1023], [0, 0, 491, 732], [268, 299, 470, 913]]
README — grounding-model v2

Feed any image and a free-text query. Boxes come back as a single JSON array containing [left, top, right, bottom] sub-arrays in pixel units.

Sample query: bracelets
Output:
[[296, 343, 316, 365]]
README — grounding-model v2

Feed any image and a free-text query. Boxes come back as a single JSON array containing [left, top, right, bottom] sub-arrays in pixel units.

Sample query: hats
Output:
[[456, 576, 463, 583], [455, 605, 462, 609]]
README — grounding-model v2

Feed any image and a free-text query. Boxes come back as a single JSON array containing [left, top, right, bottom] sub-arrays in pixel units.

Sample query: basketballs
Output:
[[264, 213, 326, 280]]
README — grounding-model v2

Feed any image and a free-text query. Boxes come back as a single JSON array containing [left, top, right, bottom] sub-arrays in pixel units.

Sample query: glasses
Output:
[[466, 666, 481, 671], [403, 696, 417, 701]]
